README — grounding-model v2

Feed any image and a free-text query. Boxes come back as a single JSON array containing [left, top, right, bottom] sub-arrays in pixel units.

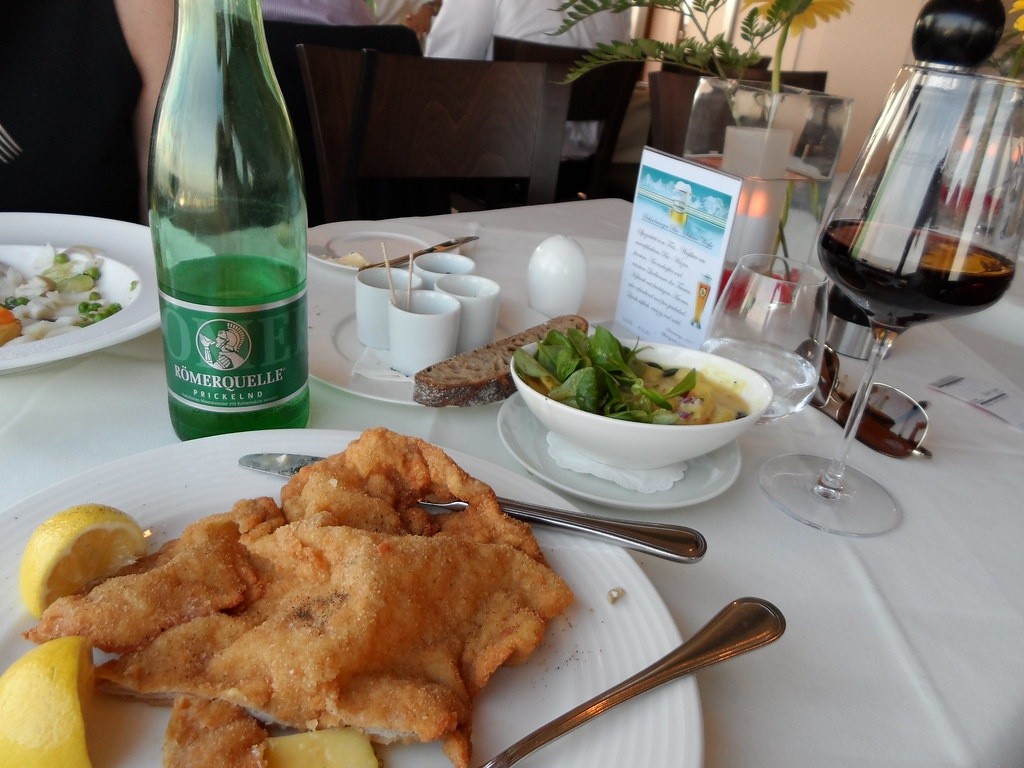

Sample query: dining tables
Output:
[[0, 195, 1024, 768]]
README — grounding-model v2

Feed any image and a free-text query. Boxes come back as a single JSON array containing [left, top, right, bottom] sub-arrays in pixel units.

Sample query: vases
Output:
[[682, 75, 849, 261]]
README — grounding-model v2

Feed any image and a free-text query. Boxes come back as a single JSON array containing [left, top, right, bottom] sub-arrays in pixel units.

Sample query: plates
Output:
[[497, 389, 742, 511], [2, 430, 702, 767], [0, 212, 162, 374], [307, 293, 551, 408], [307, 220, 460, 270]]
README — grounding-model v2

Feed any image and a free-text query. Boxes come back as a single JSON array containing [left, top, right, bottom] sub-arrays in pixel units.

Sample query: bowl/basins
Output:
[[510, 336, 773, 471]]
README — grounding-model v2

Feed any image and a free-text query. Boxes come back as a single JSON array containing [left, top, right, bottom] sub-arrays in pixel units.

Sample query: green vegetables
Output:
[[512, 324, 700, 425]]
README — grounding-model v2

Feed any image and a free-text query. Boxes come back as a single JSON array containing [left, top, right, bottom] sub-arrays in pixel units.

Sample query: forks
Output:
[[0, 124, 22, 163]]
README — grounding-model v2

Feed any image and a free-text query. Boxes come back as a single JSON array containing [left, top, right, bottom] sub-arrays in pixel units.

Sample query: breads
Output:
[[412, 314, 589, 407]]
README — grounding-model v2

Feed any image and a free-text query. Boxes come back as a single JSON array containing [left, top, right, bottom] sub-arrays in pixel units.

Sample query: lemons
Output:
[[0, 634, 93, 768], [20, 504, 150, 619]]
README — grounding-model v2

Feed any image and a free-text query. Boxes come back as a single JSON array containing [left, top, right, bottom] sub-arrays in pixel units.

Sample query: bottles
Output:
[[149, 1, 309, 440]]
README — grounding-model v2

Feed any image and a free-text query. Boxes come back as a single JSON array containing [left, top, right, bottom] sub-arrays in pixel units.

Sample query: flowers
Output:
[[541, 0, 850, 90]]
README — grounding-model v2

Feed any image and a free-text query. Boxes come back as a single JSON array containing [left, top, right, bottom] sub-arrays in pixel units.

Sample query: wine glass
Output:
[[757, 60, 1024, 537]]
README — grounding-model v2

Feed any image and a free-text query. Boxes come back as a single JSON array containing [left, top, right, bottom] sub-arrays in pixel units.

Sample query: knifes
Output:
[[359, 236, 481, 272], [240, 452, 707, 568]]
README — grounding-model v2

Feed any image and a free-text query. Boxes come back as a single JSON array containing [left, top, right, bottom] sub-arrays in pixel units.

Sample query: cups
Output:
[[697, 253, 829, 420], [387, 290, 460, 377], [412, 252, 476, 291], [435, 275, 502, 356], [355, 266, 423, 352]]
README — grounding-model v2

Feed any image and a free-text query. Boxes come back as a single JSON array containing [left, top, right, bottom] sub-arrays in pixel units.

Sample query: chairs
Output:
[[294, 42, 826, 221]]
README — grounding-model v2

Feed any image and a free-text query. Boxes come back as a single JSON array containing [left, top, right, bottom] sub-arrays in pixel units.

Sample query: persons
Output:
[[215, 0, 442, 53], [423, 0, 631, 161], [0, 0, 239, 236]]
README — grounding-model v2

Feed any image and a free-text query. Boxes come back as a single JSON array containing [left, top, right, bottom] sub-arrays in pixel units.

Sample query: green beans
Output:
[[5, 253, 122, 325]]
